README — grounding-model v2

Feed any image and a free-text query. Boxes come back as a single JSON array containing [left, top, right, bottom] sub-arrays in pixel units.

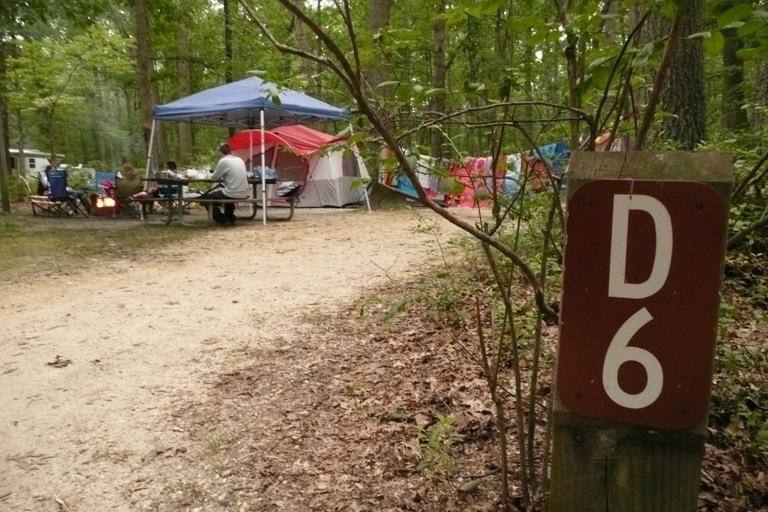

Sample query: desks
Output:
[[142, 175, 276, 227]]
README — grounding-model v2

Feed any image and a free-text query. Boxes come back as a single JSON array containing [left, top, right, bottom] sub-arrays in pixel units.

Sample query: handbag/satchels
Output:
[[275, 181, 300, 197]]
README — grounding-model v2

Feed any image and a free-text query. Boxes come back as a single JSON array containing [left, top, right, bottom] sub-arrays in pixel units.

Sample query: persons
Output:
[[153, 161, 178, 206], [112, 162, 142, 208], [198, 143, 249, 224], [37, 165, 84, 198]]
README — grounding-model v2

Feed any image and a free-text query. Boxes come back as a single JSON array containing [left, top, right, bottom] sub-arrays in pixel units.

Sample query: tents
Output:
[[225, 124, 372, 208]]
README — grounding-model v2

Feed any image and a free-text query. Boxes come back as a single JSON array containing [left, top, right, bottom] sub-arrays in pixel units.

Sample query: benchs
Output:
[[128, 196, 298, 227]]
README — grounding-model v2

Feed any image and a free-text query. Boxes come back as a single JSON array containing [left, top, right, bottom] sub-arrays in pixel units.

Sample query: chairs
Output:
[[46, 168, 118, 218]]
